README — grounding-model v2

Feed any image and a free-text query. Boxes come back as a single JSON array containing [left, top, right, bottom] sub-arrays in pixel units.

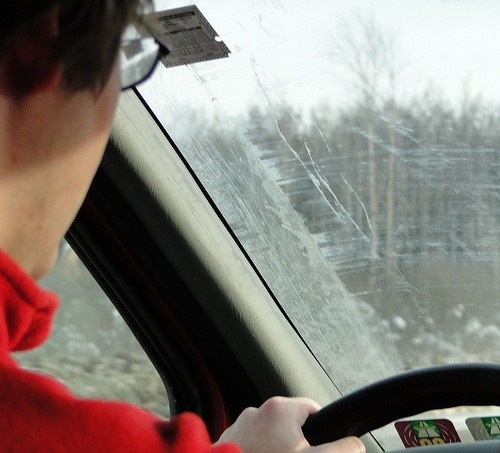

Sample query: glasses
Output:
[[120, 3, 170, 91]]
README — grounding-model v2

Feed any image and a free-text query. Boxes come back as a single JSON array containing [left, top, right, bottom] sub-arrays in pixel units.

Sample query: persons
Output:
[[0, 0, 367, 452]]
